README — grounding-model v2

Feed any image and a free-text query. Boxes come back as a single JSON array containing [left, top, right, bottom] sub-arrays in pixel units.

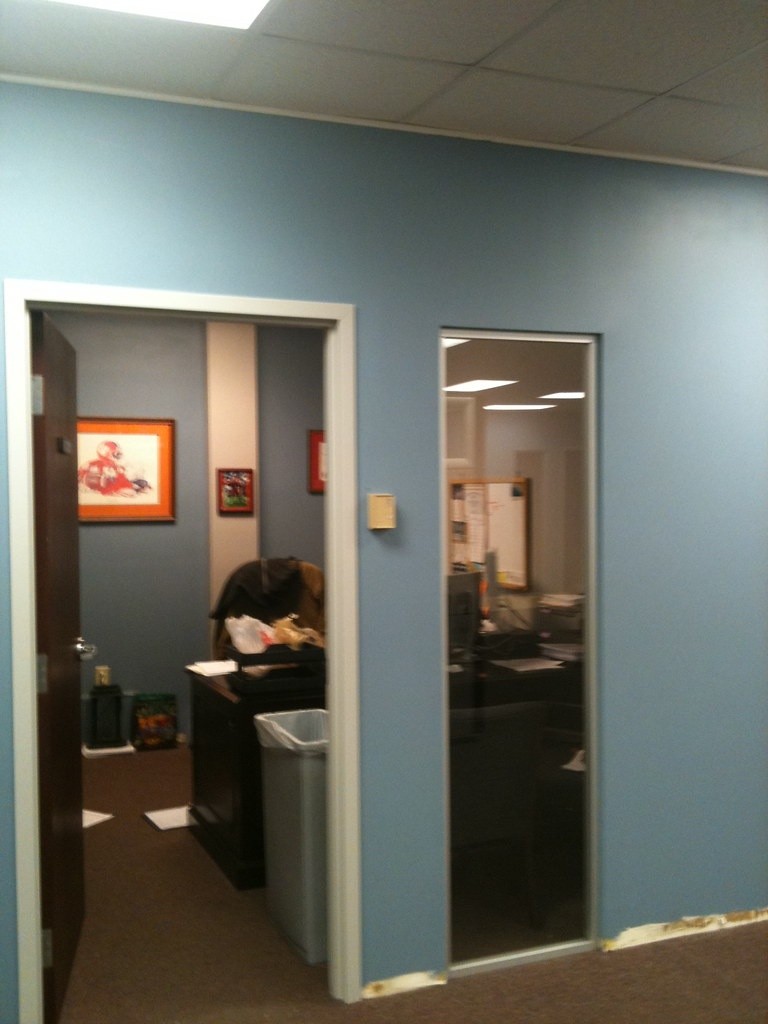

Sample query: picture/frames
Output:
[[216, 466, 255, 516], [306, 430, 326, 496], [76, 417, 179, 525]]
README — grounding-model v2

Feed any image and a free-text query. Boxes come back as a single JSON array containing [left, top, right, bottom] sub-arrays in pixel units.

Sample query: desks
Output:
[[183, 631, 584, 890]]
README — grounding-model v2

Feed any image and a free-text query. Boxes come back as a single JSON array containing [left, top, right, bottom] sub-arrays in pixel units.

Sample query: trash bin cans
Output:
[[249, 708, 340, 966]]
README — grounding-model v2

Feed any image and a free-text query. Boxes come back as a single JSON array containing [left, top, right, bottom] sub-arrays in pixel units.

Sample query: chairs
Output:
[[448, 657, 567, 853]]
